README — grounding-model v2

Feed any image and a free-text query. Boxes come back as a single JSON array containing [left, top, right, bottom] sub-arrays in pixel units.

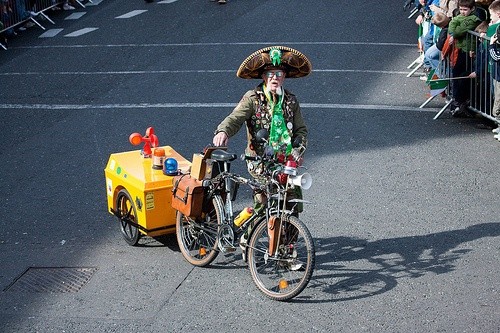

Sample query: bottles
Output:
[[233, 207, 253, 226]]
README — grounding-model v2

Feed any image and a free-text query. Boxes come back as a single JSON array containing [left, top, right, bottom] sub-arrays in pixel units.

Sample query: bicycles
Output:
[[175, 128, 325, 300]]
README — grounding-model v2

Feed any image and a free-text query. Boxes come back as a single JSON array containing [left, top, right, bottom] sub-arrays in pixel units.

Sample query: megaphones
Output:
[[288, 172, 312, 190]]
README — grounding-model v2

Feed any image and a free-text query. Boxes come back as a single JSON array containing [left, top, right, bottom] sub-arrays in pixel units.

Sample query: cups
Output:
[[153, 148, 165, 169]]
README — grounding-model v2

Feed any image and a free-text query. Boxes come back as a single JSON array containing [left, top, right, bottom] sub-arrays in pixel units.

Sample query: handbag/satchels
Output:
[[171, 174, 204, 217]]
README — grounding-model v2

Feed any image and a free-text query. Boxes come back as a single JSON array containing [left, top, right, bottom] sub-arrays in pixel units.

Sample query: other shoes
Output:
[[419, 76, 427, 81], [240, 233, 255, 261], [492, 127, 500, 141], [27, 22, 35, 27], [64, 4, 75, 10], [278, 258, 306, 270], [19, 27, 26, 30]]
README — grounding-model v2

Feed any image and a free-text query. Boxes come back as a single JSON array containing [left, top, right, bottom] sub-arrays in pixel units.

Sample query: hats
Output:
[[237, 45, 312, 79]]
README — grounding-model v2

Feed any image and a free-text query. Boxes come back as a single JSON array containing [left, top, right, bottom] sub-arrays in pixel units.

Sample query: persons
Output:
[[0, 0, 75, 45], [403, 0, 500, 142], [212, 46, 312, 271]]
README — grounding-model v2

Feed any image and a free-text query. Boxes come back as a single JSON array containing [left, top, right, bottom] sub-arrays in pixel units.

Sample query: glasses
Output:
[[265, 71, 284, 78]]
